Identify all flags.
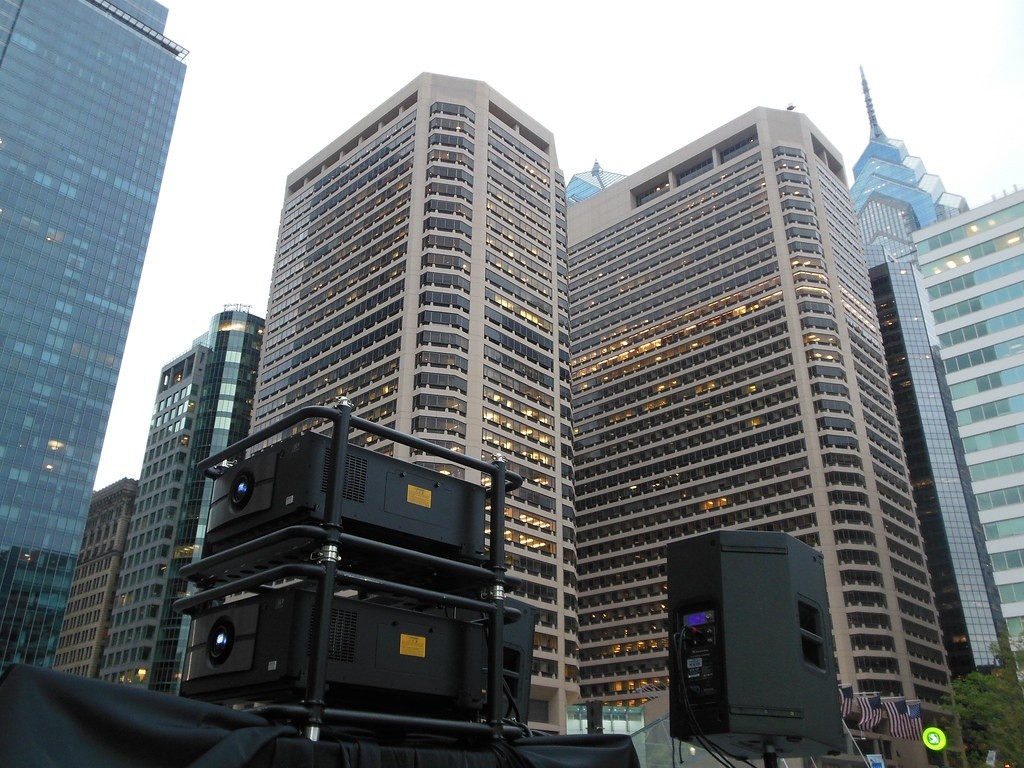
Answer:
[883,699,913,738]
[902,702,923,741]
[838,686,853,718]
[856,695,882,730]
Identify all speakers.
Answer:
[666,529,846,759]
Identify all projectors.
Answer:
[204,427,486,595]
[179,581,487,724]
